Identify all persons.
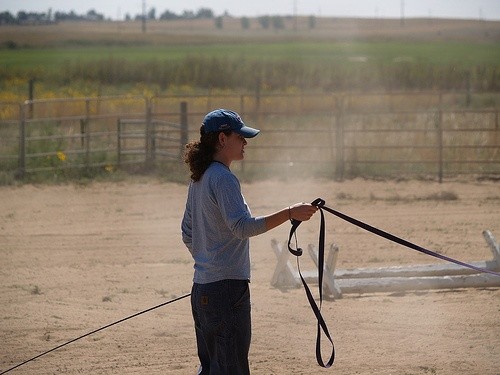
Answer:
[180,109,318,375]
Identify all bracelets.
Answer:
[287,206,293,219]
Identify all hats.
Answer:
[201,108,261,138]
